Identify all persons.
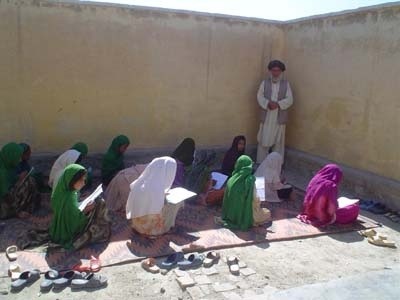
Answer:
[170,138,203,190]
[125,156,184,236]
[253,152,296,203]
[256,60,294,165]
[0,142,37,218]
[296,164,360,229]
[47,164,113,252]
[45,142,92,190]
[214,154,272,229]
[102,164,149,217]
[222,136,247,178]
[101,135,136,187]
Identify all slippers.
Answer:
[161,252,184,268]
[359,200,400,222]
[226,255,240,274]
[71,258,101,272]
[362,229,387,240]
[40,269,59,291]
[368,236,396,247]
[203,251,220,267]
[177,254,206,268]
[10,270,39,289]
[6,245,18,261]
[53,270,85,289]
[71,273,108,289]
[141,258,160,273]
[10,265,20,280]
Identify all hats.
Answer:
[268,60,285,72]
